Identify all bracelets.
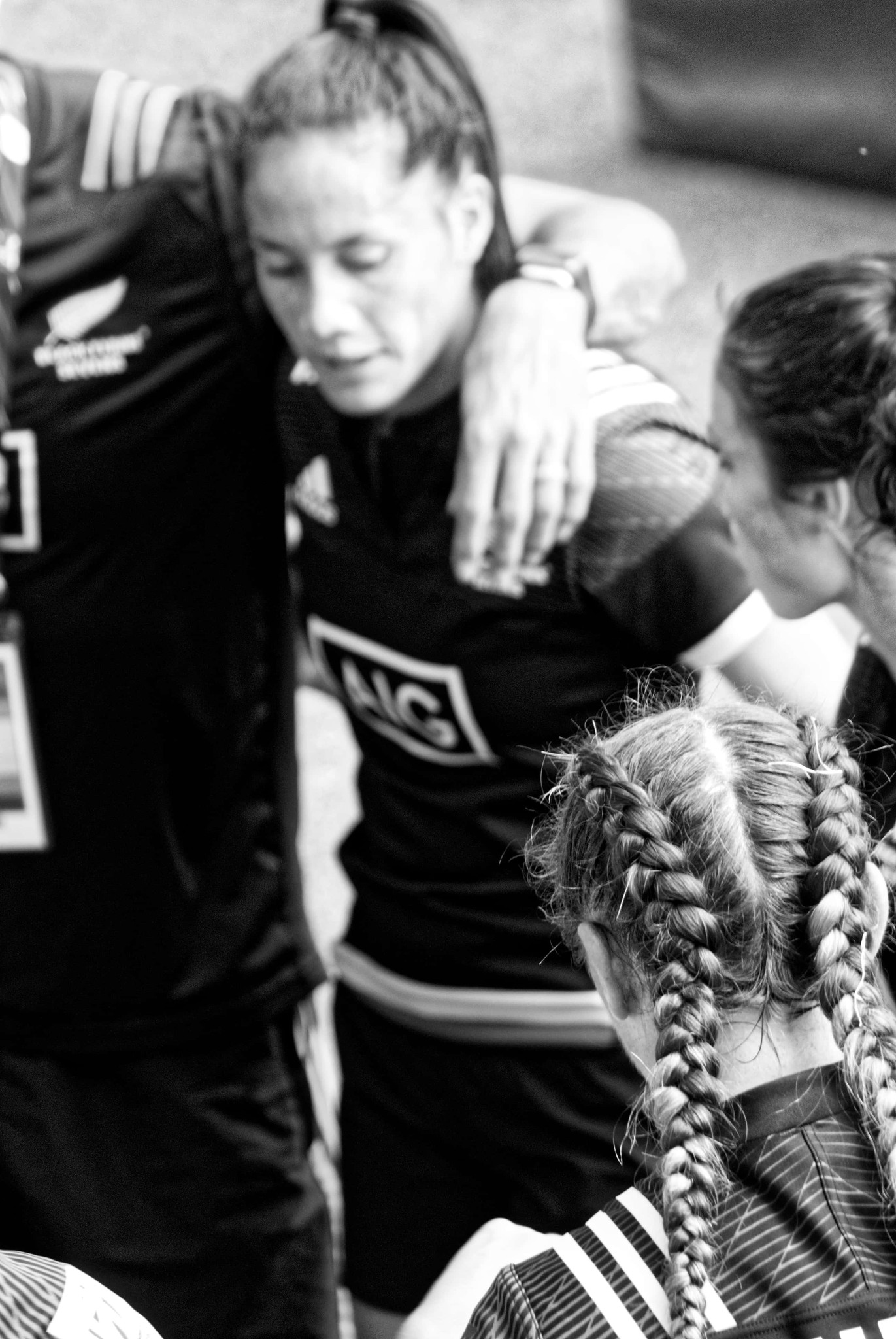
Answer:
[510,244,597,340]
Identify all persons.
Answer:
[232,0,848,1339]
[0,54,689,1339]
[708,255,896,829]
[451,696,896,1339]
